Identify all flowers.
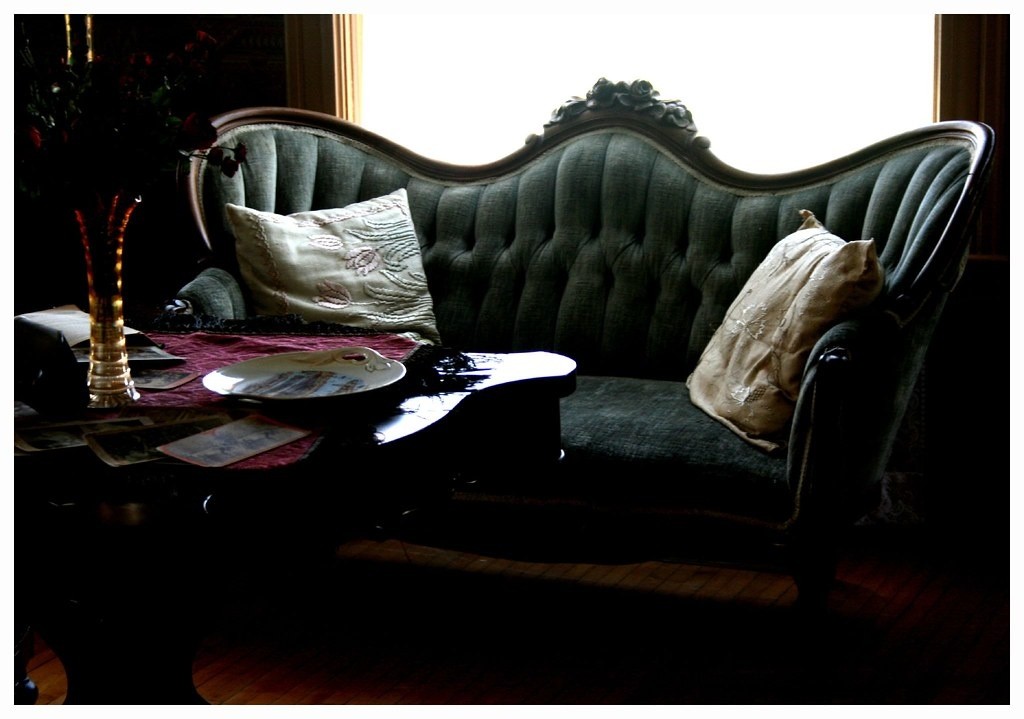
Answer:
[15,26,247,200]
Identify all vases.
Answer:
[69,193,147,407]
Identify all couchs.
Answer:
[147,79,996,705]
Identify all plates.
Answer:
[202,346,408,404]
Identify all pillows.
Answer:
[224,188,443,345]
[686,208,886,451]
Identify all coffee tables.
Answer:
[15,351,578,705]
